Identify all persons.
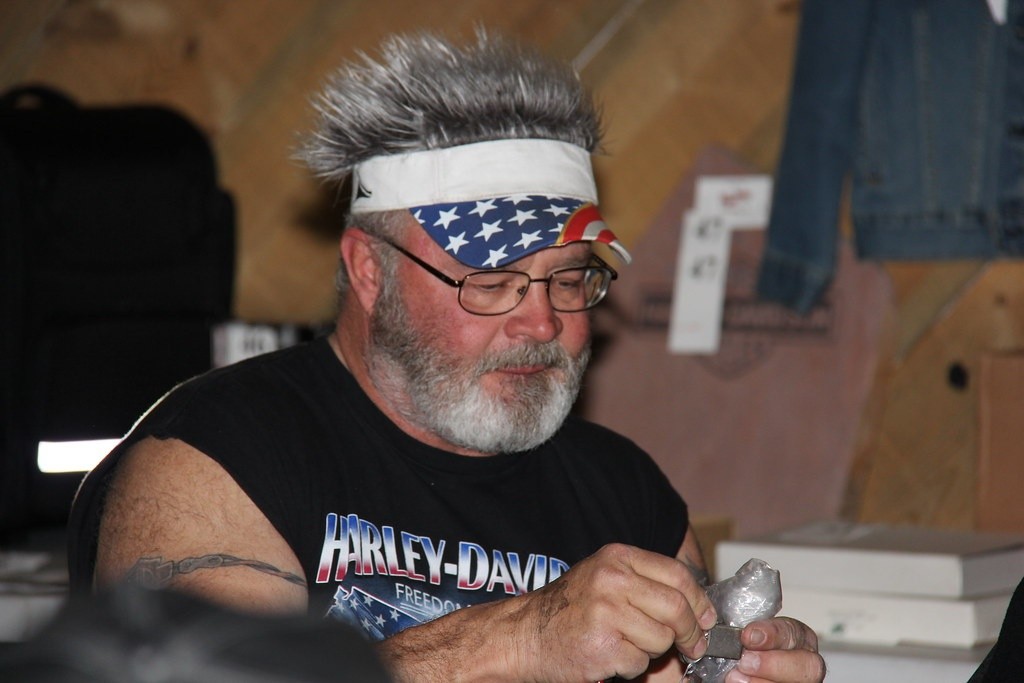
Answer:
[66,22,826,682]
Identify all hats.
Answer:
[349,136,633,271]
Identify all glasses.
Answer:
[356,225,619,317]
